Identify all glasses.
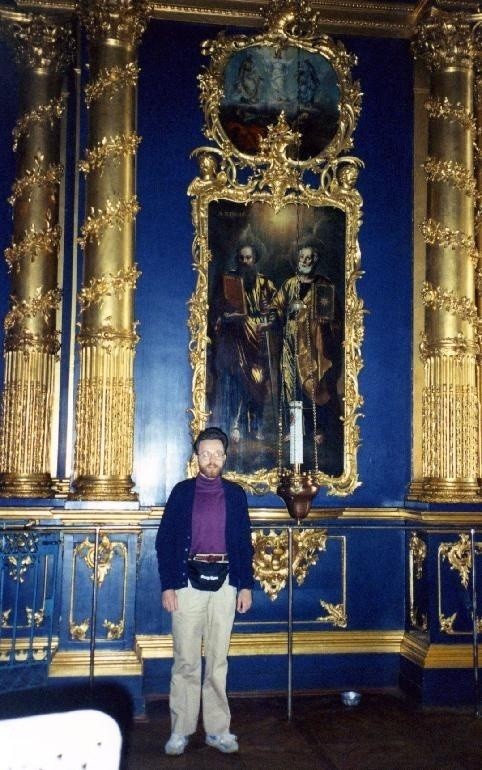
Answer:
[197,449,225,461]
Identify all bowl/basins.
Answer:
[341,690,362,706]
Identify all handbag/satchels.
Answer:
[185,559,229,591]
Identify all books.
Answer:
[215,270,247,313]
[298,281,336,320]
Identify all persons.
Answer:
[152,426,256,756]
[234,46,320,105]
[214,232,278,444]
[254,236,340,445]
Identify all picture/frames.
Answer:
[183,30,366,171]
[198,105,369,493]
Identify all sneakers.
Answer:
[204,732,241,755]
[165,733,190,756]
